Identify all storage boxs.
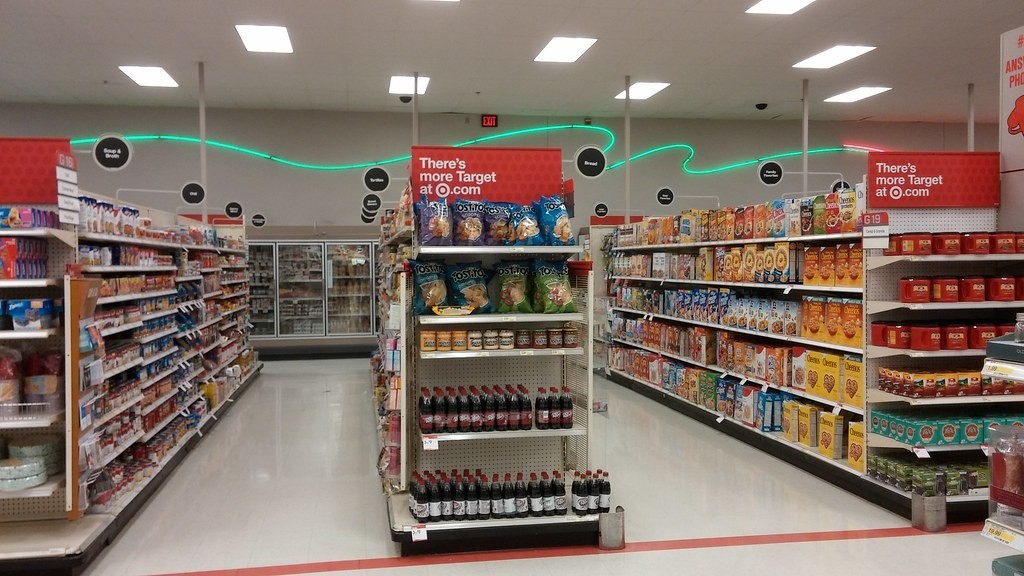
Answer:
[804,241,863,287]
[796,241,804,285]
[785,302,801,339]
[818,411,844,460]
[800,295,863,349]
[798,404,817,447]
[784,182,863,236]
[792,346,810,392]
[805,350,821,397]
[782,400,798,442]
[602,199,784,433]
[842,359,863,408]
[385,305,402,410]
[0,298,65,331]
[847,421,864,473]
[820,352,845,403]
[806,404,823,446]
[774,242,797,283]
[0,207,60,279]
[783,348,792,387]
[868,365,1024,497]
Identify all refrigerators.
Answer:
[253,237,379,356]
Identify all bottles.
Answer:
[571,469,612,516]
[491,472,567,519]
[408,467,489,525]
[419,384,532,434]
[536,386,572,429]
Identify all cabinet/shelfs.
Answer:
[328,256,370,317]
[0,204,117,576]
[598,232,867,496]
[280,258,322,322]
[386,245,602,558]
[979,358,1024,553]
[248,260,274,323]
[79,189,263,536]
[859,173,1024,526]
